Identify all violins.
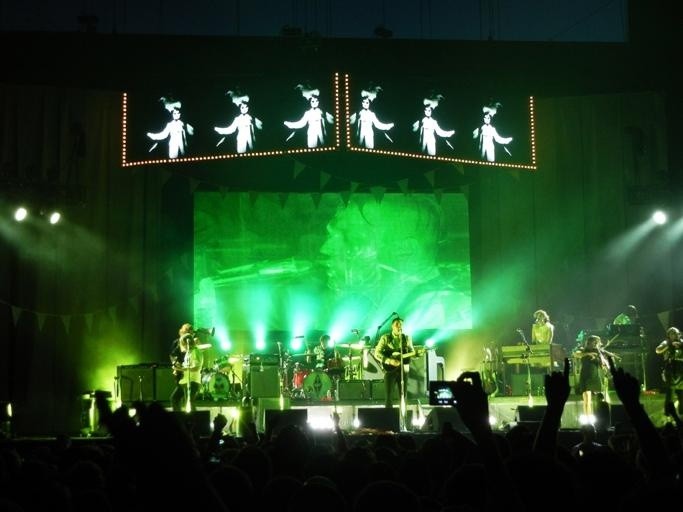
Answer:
[592,348,621,362]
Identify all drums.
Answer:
[326,359,344,373]
[294,367,311,390]
[212,357,232,375]
[200,368,216,383]
[201,372,230,401]
[302,372,335,401]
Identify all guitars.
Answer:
[381,345,438,372]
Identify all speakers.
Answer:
[371,380,399,402]
[425,408,470,433]
[339,380,371,401]
[358,409,399,432]
[611,404,644,423]
[170,411,210,436]
[607,348,647,391]
[117,363,177,406]
[251,353,280,402]
[266,409,307,436]
[516,406,547,421]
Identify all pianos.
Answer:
[501,344,561,374]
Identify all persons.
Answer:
[214,102,263,153]
[413,105,455,156]
[284,96,334,148]
[350,97,394,148]
[473,113,513,163]
[147,108,195,158]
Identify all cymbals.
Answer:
[341,343,362,348]
[342,356,361,360]
[294,353,318,356]
[195,344,211,349]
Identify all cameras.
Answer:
[429,381,471,406]
